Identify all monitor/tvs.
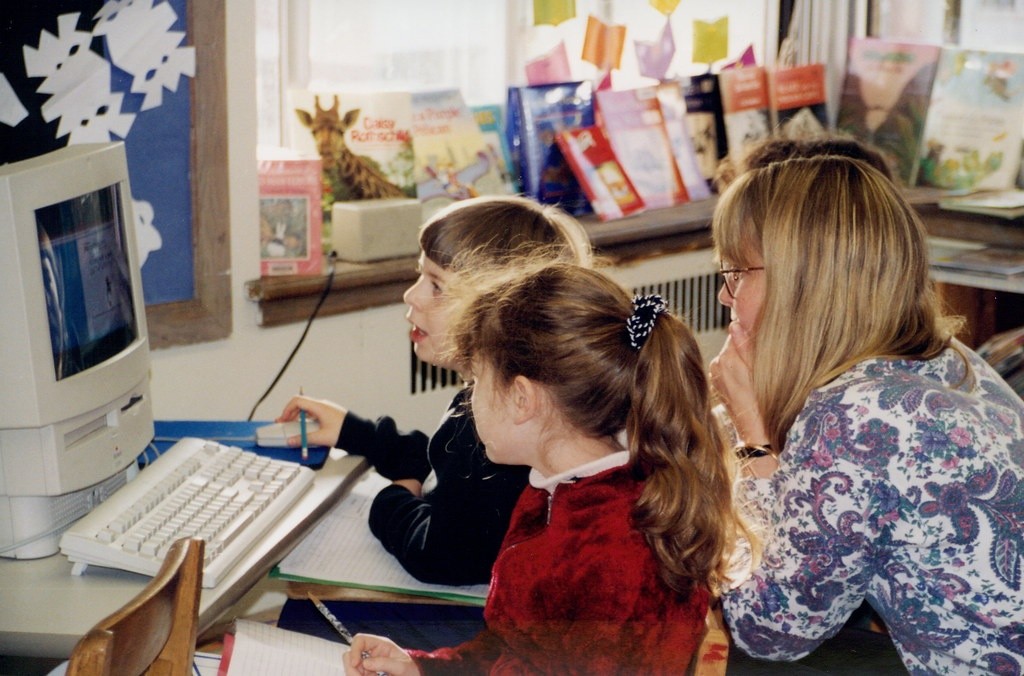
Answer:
[1,138,157,560]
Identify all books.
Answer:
[345,63,829,221]
[838,38,1024,279]
[271,473,492,606]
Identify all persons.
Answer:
[275,200,586,654]
[708,156,1024,675]
[745,140,891,180]
[343,264,758,676]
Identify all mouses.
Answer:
[255,419,322,449]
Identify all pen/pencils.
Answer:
[297,387,308,460]
[306,589,383,675]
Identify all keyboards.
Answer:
[59,435,316,592]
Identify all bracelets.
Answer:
[737,444,772,457]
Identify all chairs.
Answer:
[63,537,205,676]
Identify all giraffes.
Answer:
[292,95,410,202]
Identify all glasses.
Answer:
[720,259,764,299]
